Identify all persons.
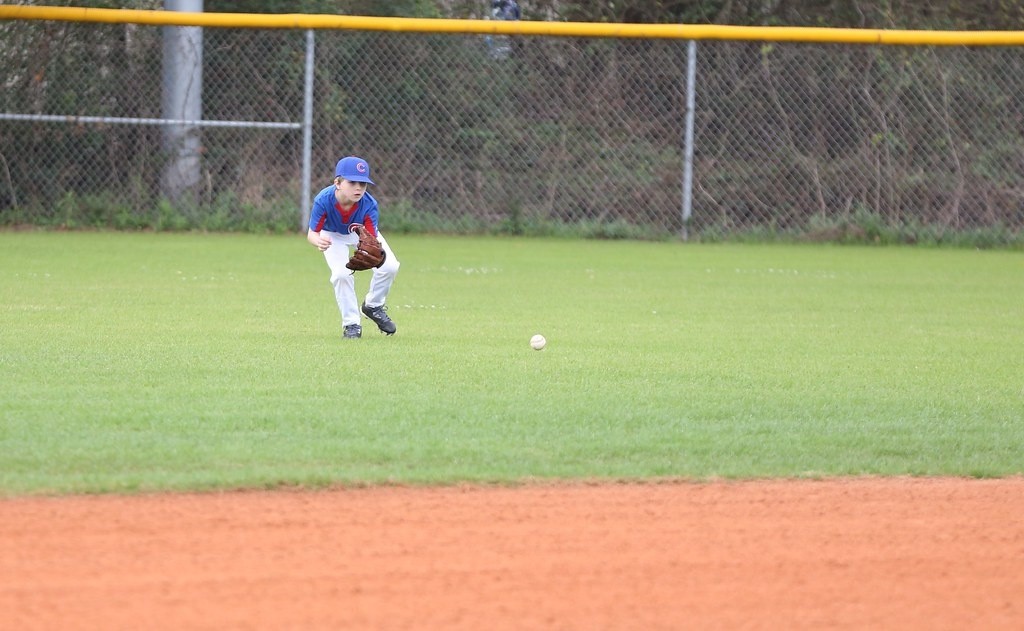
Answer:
[306,155,400,338]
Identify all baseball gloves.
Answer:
[344,225,387,272]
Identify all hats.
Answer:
[334,155,376,186]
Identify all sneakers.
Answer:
[343,324,362,339]
[360,300,396,336]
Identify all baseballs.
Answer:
[530,334,547,350]
